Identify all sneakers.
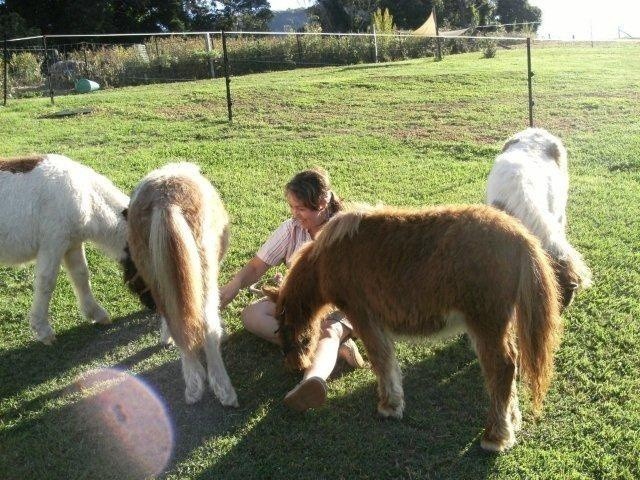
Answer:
[338,338,363,368]
[283,375,327,413]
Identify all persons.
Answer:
[219,170,364,413]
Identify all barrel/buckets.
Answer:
[75,79,101,93]
